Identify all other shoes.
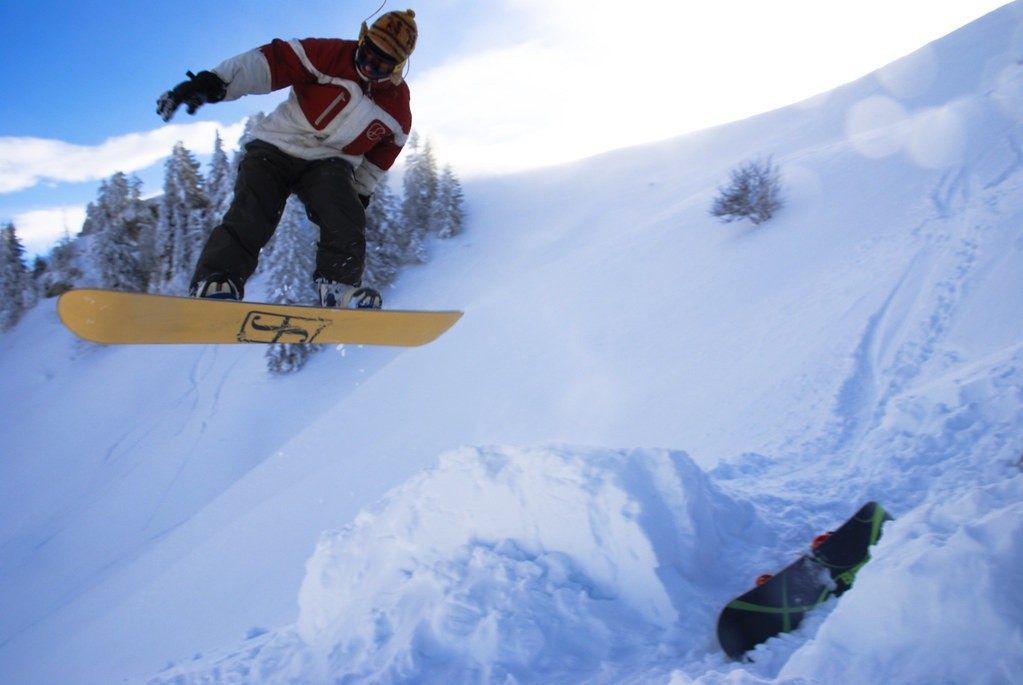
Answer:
[190,274,243,301]
[311,278,383,309]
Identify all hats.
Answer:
[359,9,418,73]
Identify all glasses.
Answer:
[355,47,393,79]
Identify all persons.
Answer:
[155,8,418,313]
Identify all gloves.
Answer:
[359,194,371,209]
[156,70,227,123]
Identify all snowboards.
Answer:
[57,287,462,347]
[718,499,896,665]
